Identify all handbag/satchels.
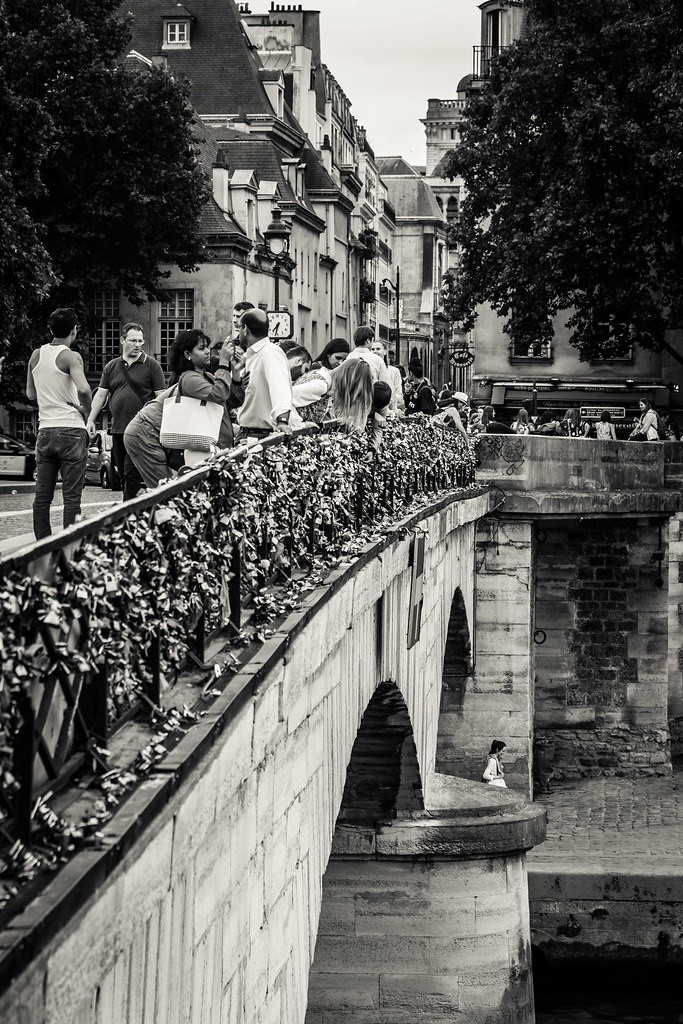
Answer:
[160,370,224,451]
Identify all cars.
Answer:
[84,429,114,490]
[0,432,62,483]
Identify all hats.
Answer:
[451,392,469,405]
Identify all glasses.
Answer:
[126,339,145,345]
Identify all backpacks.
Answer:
[650,412,668,441]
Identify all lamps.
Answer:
[667,382,679,393]
[625,379,635,387]
[550,377,561,385]
[479,379,492,388]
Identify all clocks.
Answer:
[265,310,294,340]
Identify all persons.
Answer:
[24,424,36,447]
[86,301,682,506]
[26,306,91,542]
[483,740,508,787]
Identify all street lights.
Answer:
[262,205,293,342]
[379,266,401,368]
